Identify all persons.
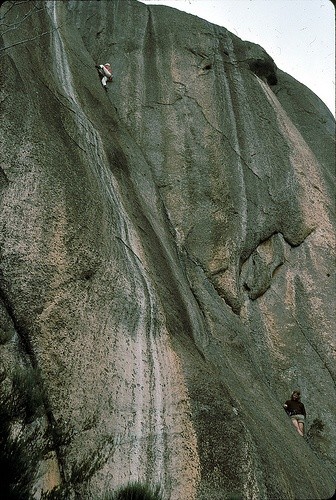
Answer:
[95,63,113,92]
[284,390,306,437]
